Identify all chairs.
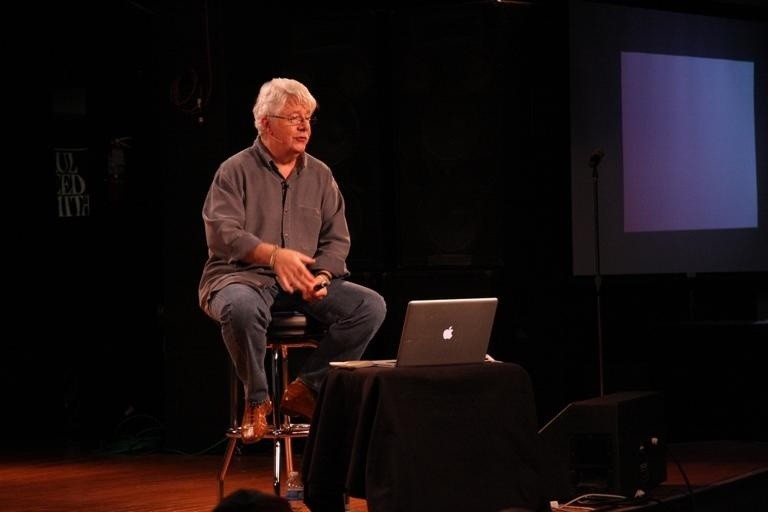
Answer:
[216,255,324,507]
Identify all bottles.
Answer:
[284,470,305,512]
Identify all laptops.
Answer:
[373,297,497,367]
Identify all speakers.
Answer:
[540,390,665,502]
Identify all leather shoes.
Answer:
[238,396,274,447]
[276,379,318,423]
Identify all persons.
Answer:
[196,74,388,445]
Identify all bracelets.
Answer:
[269,244,279,270]
[315,272,332,281]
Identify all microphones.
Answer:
[588,147,605,169]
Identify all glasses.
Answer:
[262,113,318,128]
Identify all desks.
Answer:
[317,358,537,510]
